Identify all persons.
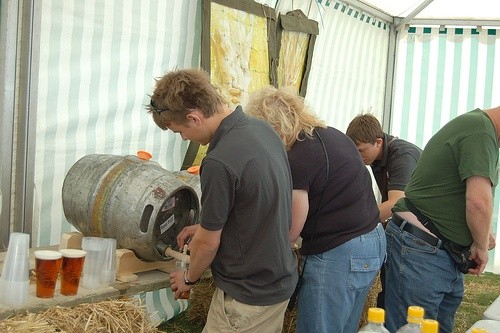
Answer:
[380,107,500,333]
[244,86,388,333]
[345,114,422,310]
[143,65,299,333]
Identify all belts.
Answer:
[392,213,445,250]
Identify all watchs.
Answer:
[183,269,200,286]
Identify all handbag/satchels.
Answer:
[444,242,477,274]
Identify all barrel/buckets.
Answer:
[61,152,202,262]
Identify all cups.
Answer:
[80,236,117,290]
[0,232,30,307]
[174,289,190,299]
[60,250,86,297]
[34,249,62,298]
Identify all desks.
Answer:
[0,246,213,322]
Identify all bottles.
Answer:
[395,305,424,333]
[421,319,439,333]
[357,307,390,333]
[470,327,489,333]
